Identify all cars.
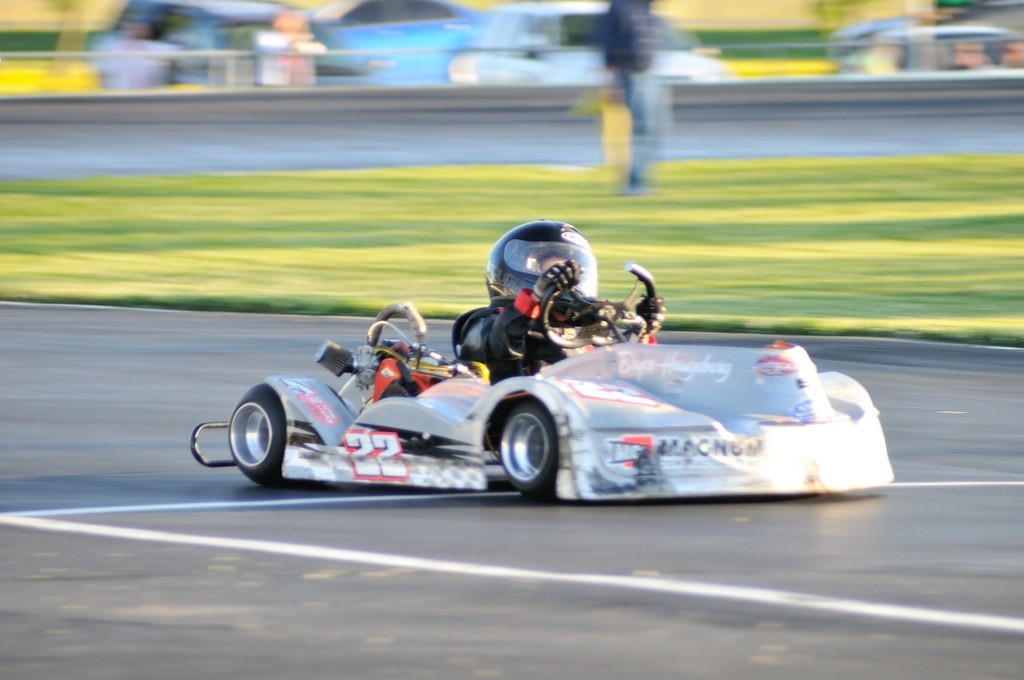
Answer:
[85,0,732,87]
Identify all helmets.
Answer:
[485,218,600,302]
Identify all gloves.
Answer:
[636,295,666,339]
[531,259,584,301]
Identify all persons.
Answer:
[254,11,326,86]
[605,0,671,195]
[452,220,666,386]
[89,9,182,90]
